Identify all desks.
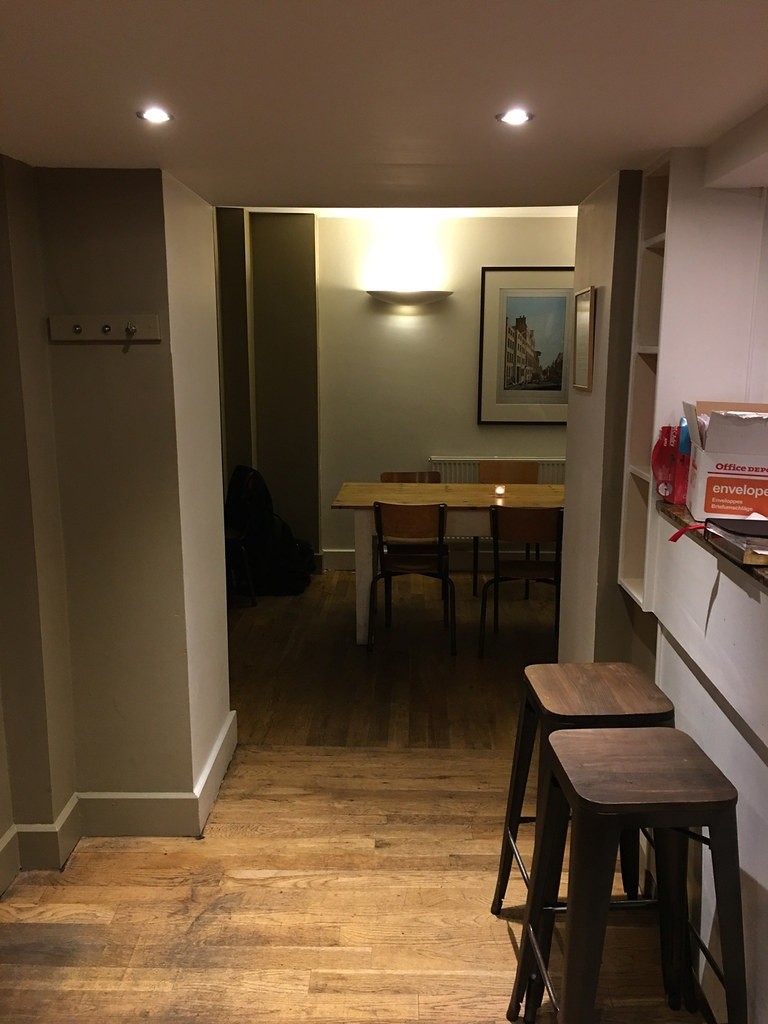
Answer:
[332,482,563,648]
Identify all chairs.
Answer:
[503,727,737,1024]
[480,503,567,645]
[466,459,544,600]
[365,501,450,650]
[491,659,678,937]
[369,470,446,623]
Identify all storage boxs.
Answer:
[687,402,768,521]
[652,426,687,504]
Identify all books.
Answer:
[704,517,768,565]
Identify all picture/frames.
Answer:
[571,285,595,392]
[477,267,573,425]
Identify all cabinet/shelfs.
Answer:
[618,195,666,612]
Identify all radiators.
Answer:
[428,455,566,542]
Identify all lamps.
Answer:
[356,285,460,307]
[134,106,174,128]
[492,101,530,132]
